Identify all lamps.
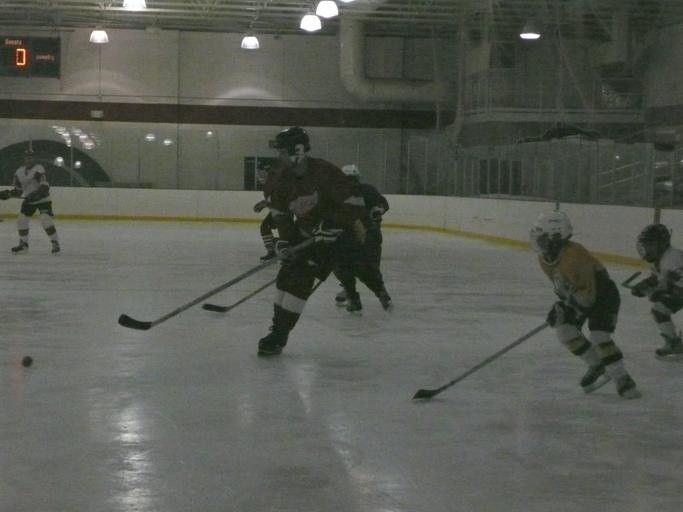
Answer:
[316,0,339,19]
[519,17,541,40]
[122,0,146,10]
[240,29,260,50]
[88,26,109,44]
[299,8,321,31]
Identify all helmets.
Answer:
[529,209,573,265]
[25,150,34,156]
[636,223,671,263]
[270,127,310,156]
[257,159,274,173]
[342,165,360,178]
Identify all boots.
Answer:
[257,302,300,354]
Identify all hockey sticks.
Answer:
[622,271,675,306]
[202,275,275,312]
[119,260,277,330]
[411,320,550,398]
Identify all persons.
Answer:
[253,159,294,260]
[11,148,61,253]
[257,127,369,354]
[334,164,391,311]
[529,209,637,401]
[631,224,683,360]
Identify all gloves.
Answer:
[0,190,12,199]
[27,191,39,202]
[254,200,267,212]
[547,300,578,327]
[632,280,651,298]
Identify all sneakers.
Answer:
[579,363,606,387]
[51,240,60,253]
[335,289,391,312]
[12,240,29,252]
[615,374,636,396]
[260,250,277,260]
[655,337,681,357]
